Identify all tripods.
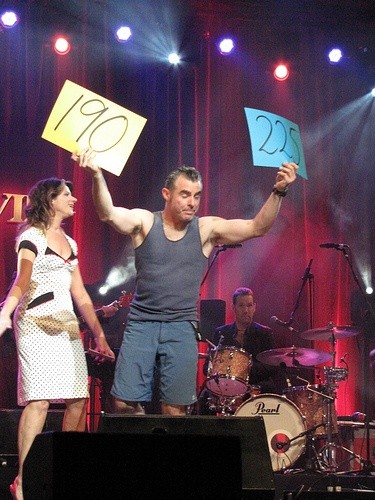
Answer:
[293,356,369,472]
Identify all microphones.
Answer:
[216,244,242,248]
[339,353,348,363]
[280,362,292,388]
[320,243,348,249]
[276,442,283,449]
[270,316,295,332]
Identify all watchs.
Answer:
[272,186,289,197]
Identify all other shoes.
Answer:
[10,476,19,500]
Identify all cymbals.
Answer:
[300,324,364,340]
[255,347,333,367]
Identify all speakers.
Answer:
[0,409,276,500]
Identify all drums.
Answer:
[283,382,339,438]
[216,384,258,416]
[204,344,251,399]
[324,368,346,381]
[233,392,306,473]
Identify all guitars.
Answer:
[76,289,134,326]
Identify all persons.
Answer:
[202,288,276,391]
[0,178,115,500]
[70,147,299,418]
